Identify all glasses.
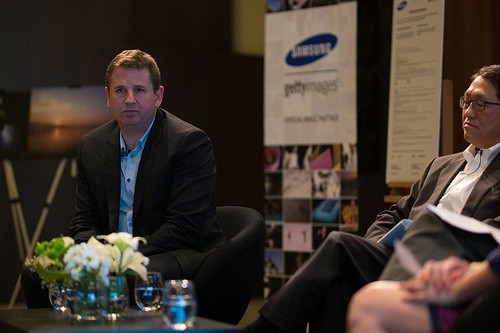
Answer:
[459,97,500,111]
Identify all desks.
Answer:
[0,308,244,333]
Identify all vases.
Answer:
[49,281,129,322]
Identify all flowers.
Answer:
[24,232,151,285]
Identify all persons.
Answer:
[347,246,500,333]
[68,50,226,309]
[223,65,500,332]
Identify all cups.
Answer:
[133,272,164,313]
[160,280,197,331]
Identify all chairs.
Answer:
[194,206,266,324]
